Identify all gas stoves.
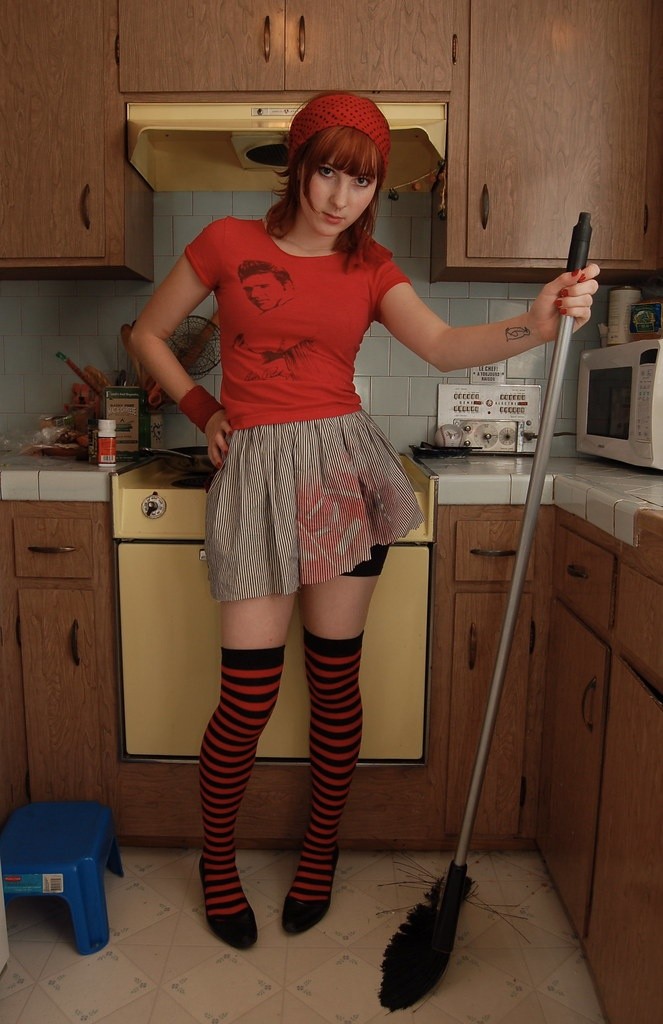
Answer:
[112,447,440,542]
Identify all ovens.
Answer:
[118,543,429,761]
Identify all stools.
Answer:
[2,800,126,956]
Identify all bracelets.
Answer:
[179,385,223,433]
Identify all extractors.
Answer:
[125,100,447,195]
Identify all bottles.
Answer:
[98,418,116,468]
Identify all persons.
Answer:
[130,90,599,950]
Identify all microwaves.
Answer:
[576,339,663,470]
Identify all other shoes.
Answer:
[195,855,257,951]
[282,847,339,933]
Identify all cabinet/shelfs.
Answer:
[430,0,663,288]
[1,499,662,1023]
[116,0,458,104]
[0,0,153,284]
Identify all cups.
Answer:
[149,413,165,448]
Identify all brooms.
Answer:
[377,206,594,1011]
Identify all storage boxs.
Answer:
[103,386,152,464]
